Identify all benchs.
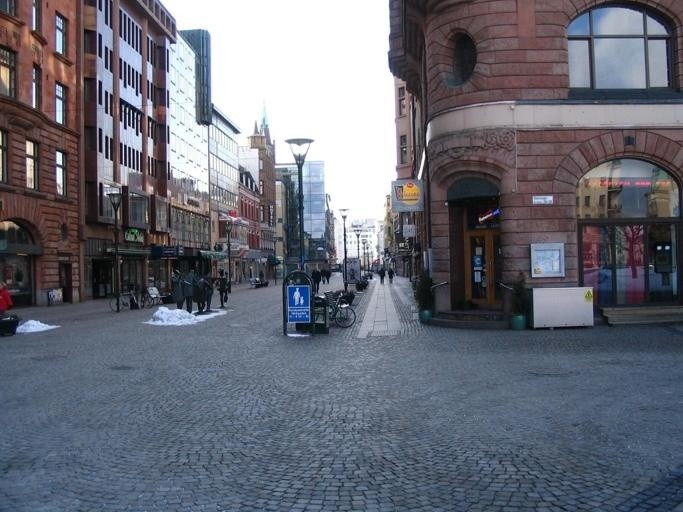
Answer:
[323,290,349,320]
[248,278,269,288]
[145,286,171,308]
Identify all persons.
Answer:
[172,267,229,313]
[259,270,265,287]
[378,268,395,285]
[310,265,331,294]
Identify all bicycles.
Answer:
[108,285,154,311]
[310,290,356,327]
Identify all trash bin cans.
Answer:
[94,282,106,297]
[309,295,329,334]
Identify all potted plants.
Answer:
[412,269,449,323]
[505,270,530,330]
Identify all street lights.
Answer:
[223,222,232,283]
[337,208,350,291]
[105,193,122,297]
[362,239,366,275]
[353,230,362,260]
[284,138,314,274]
[272,239,278,285]
[365,244,370,272]
